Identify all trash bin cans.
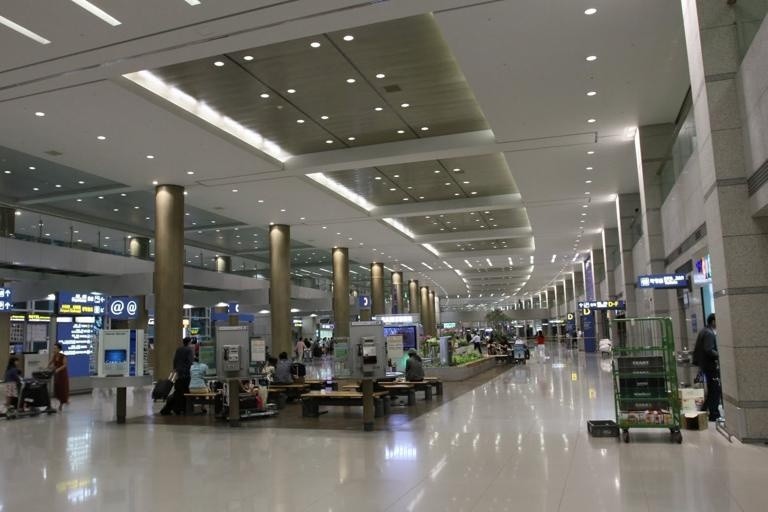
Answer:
[576,337,584,350]
[440,337,452,366]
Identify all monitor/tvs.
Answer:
[384,325,417,351]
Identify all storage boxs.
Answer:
[678,388,705,412]
[679,411,708,431]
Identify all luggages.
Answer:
[151,372,177,402]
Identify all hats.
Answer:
[408,348,417,353]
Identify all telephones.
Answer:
[223,345,240,362]
[356,337,376,356]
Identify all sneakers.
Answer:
[716,417,725,421]
[202,408,207,413]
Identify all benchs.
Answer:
[484,349,514,363]
[183,376,442,426]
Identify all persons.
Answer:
[566,327,581,349]
[44,342,69,413]
[533,331,546,365]
[466,330,524,355]
[404,348,424,382]
[293,337,332,362]
[5,357,21,386]
[692,312,724,422]
[173,336,294,417]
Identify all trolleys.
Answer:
[6,367,57,419]
[611,317,684,444]
